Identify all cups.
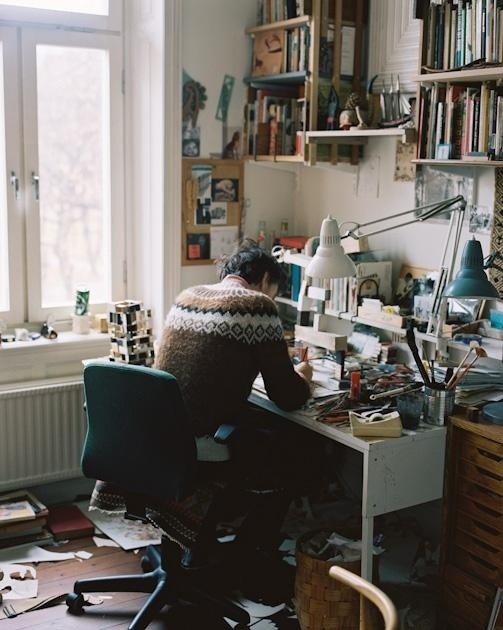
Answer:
[395,395,423,430]
[424,381,455,425]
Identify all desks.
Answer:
[249,371,447,629]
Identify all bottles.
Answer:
[74,286,89,315]
[255,218,288,249]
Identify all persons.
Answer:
[395,272,413,300]
[151,238,312,597]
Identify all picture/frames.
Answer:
[394,263,441,309]
[181,158,246,266]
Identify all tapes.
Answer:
[352,412,384,422]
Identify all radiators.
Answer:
[0,373,88,493]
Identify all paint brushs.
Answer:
[370,382,424,400]
[406,329,488,390]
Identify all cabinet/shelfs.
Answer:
[242,0,368,167]
[411,0,503,168]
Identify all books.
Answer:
[270,236,394,321]
[246,0,356,159]
[418,0,502,165]
[46,503,95,542]
[246,343,502,413]
[0,488,53,550]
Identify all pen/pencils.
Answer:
[302,347,308,361]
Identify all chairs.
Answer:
[66,364,286,630]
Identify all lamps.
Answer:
[442,235,503,301]
[305,196,467,338]
